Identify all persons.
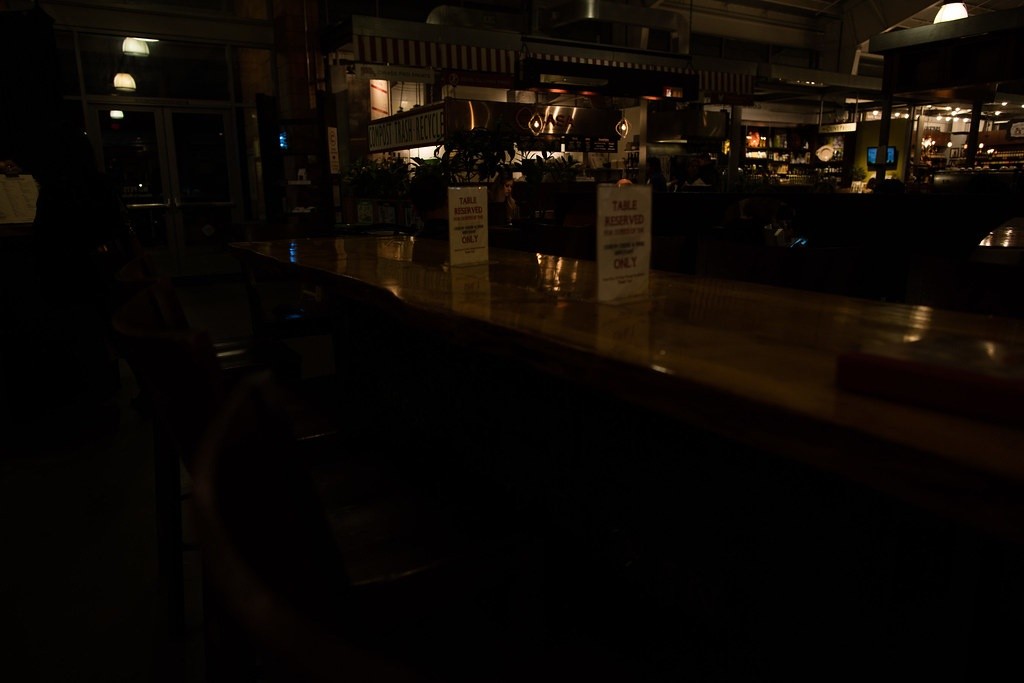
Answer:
[393,157,1024,308]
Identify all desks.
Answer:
[231,228,1023,481]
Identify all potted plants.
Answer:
[511,155,548,209]
[376,156,418,231]
[343,159,381,225]
[549,156,586,208]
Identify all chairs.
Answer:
[108,293,455,589]
